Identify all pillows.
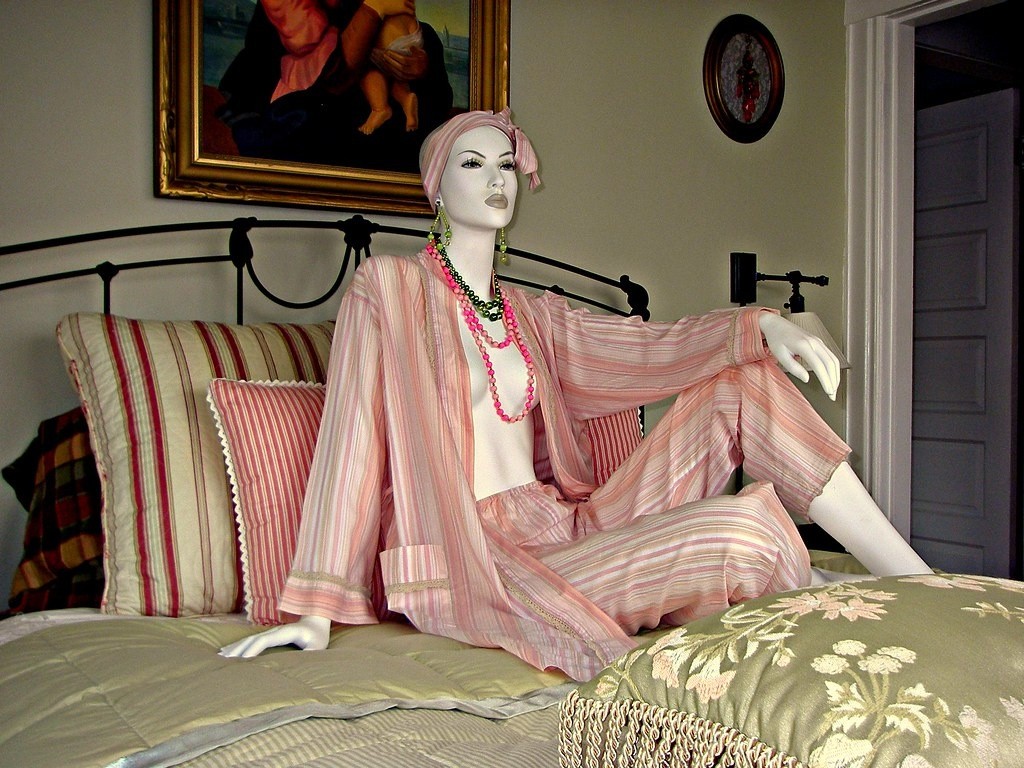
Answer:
[56,310,340,620]
[206,375,409,627]
[556,572,1024,768]
[581,404,645,491]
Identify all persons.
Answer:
[216,113,935,686]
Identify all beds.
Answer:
[0,215,848,768]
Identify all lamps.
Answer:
[728,252,854,375]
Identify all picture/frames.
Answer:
[702,13,785,144]
[151,0,512,220]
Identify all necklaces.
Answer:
[426,238,534,423]
